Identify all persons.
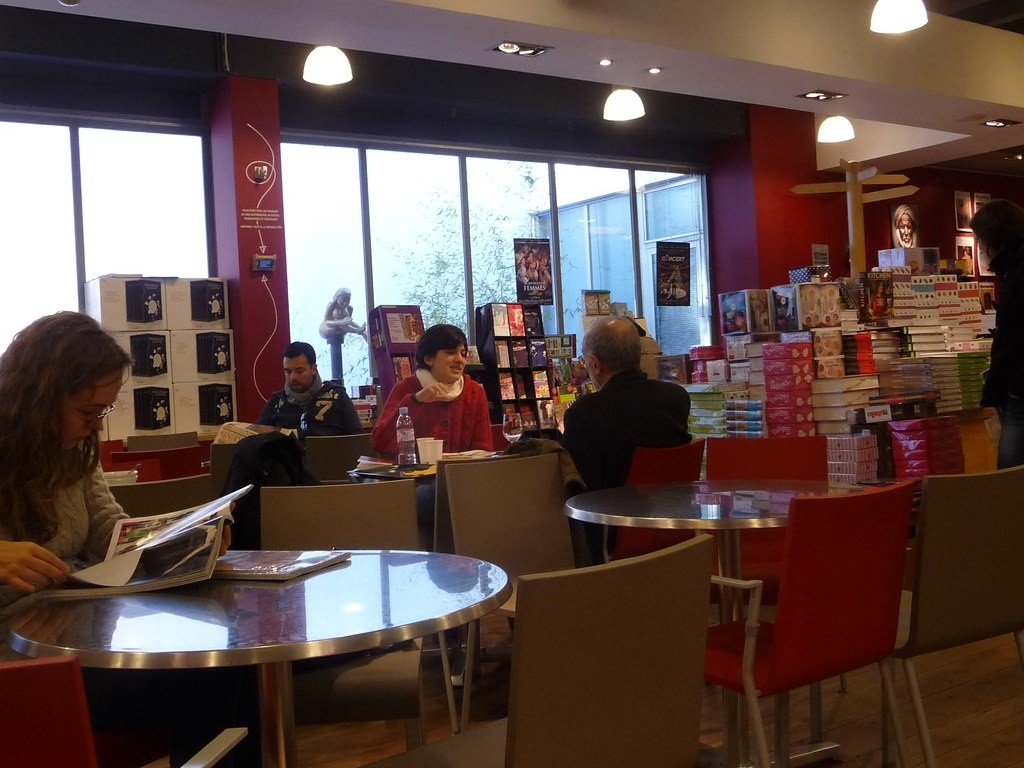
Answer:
[371,323,494,461]
[967,200,1024,470]
[0,311,262,768]
[562,316,693,566]
[868,279,892,316]
[962,246,973,275]
[256,341,364,446]
[318,287,367,341]
[515,245,551,284]
[893,205,919,249]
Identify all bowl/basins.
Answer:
[103,470,138,485]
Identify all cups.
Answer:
[502,413,523,444]
[416,437,434,464]
[425,440,443,464]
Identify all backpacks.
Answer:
[218,432,323,550]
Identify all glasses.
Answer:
[65,402,116,425]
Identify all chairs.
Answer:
[0,433,1024,768]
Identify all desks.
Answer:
[346,451,537,485]
[5,549,512,768]
[562,480,891,768]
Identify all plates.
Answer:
[442,449,497,457]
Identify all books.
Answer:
[211,550,351,581]
[42,484,254,597]
[835,271,894,324]
[743,324,992,434]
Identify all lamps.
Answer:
[870,0,929,34]
[603,84,646,122]
[817,113,855,143]
[302,44,353,85]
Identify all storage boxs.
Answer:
[654,354,708,383]
[765,373,815,392]
[877,463,965,481]
[718,282,843,334]
[107,384,175,442]
[788,265,832,284]
[173,381,238,433]
[84,277,167,331]
[169,330,235,382]
[877,426,959,441]
[879,435,962,453]
[763,341,813,360]
[688,345,722,361]
[105,331,172,385]
[850,414,959,435]
[878,448,964,463]
[768,421,817,437]
[763,358,815,376]
[164,277,229,331]
[766,406,814,424]
[764,389,811,406]
[877,455,965,469]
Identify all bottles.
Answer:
[396,407,416,465]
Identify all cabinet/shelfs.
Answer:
[473,301,560,435]
